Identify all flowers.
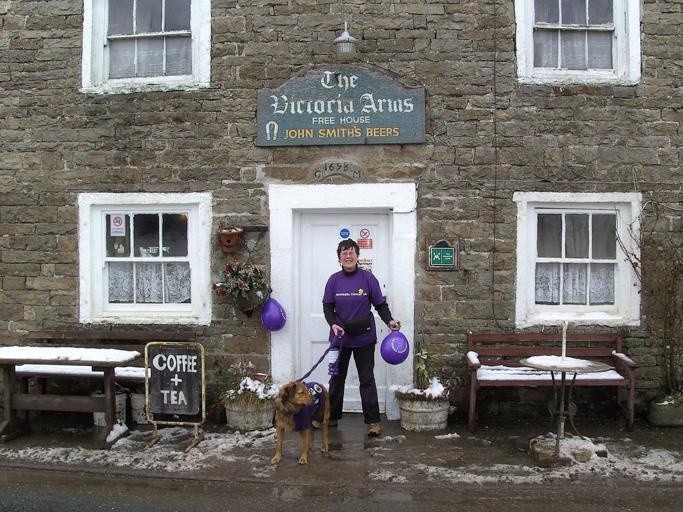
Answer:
[210,262,269,315]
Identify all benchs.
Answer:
[464,327,641,433]
[23,329,198,420]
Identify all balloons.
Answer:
[380,331,409,366]
[260,297,286,332]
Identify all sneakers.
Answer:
[327,419,339,426]
[366,424,383,436]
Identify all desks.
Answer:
[518,353,611,467]
[0,344,138,451]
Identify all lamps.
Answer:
[333,18,360,60]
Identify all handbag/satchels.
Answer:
[335,311,373,338]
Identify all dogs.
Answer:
[270,380,330,466]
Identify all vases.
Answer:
[216,223,242,253]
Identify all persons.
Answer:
[312,236,401,436]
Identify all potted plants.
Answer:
[396,336,461,432]
[213,357,274,430]
